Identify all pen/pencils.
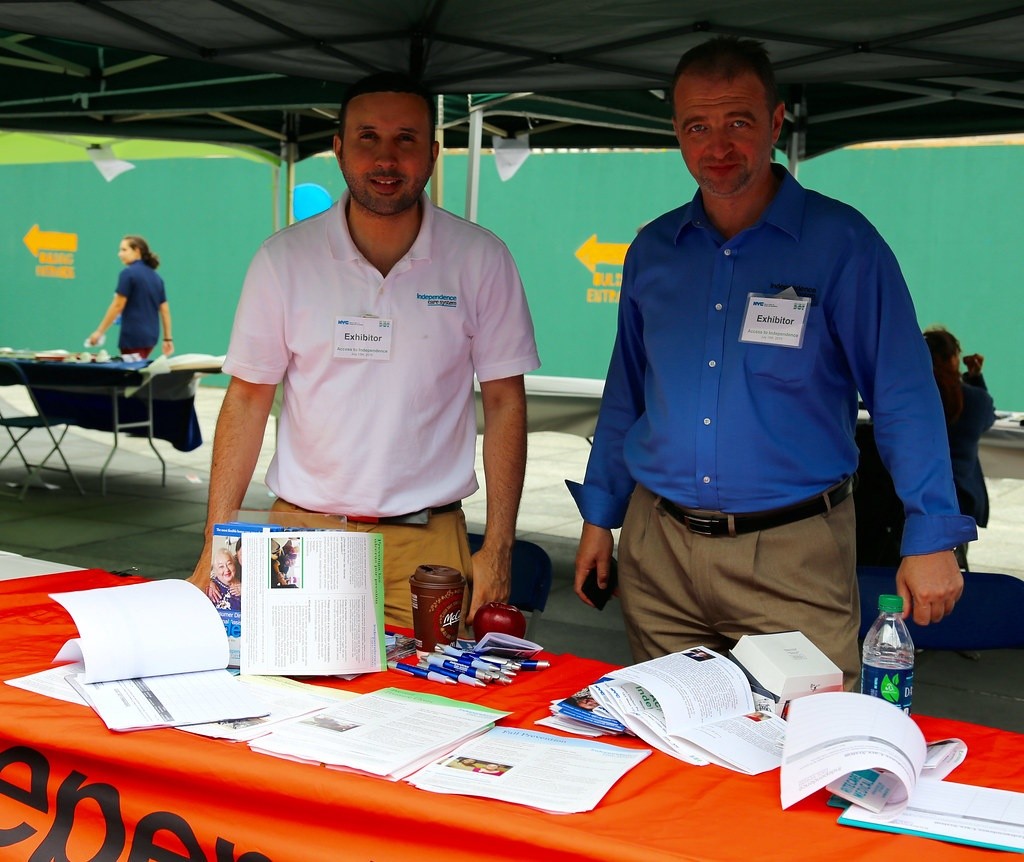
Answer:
[387,640,551,689]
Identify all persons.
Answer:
[185,72,541,636]
[576,39,977,693]
[89,235,174,360]
[922,330,995,529]
[210,538,242,609]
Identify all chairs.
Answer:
[856,565,1024,652]
[0,360,85,503]
[852,416,971,573]
[467,530,556,641]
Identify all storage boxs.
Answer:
[212,520,347,678]
[726,629,845,720]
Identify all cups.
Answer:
[410,563,467,659]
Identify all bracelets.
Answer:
[163,339,172,341]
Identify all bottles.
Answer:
[859,592,916,719]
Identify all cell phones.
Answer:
[582,556,618,611]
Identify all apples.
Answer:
[472,601,527,644]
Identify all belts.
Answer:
[651,479,854,537]
[378,498,463,526]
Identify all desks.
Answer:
[0,550,1024,862]
[0,356,223,497]
[471,374,1024,482]
